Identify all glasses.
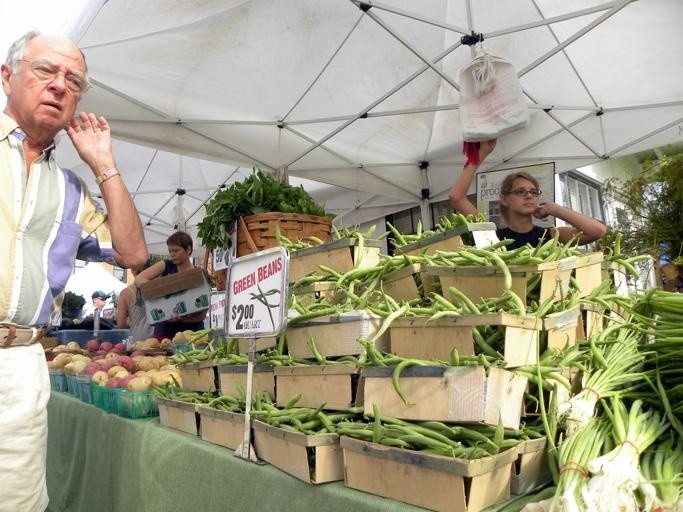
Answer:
[18,58,91,94]
[512,188,541,198]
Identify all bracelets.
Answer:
[95,168,120,184]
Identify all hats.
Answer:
[92,290,109,301]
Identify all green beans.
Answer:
[151,218,653,460]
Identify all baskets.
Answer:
[203,211,333,290]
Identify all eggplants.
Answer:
[47,315,116,335]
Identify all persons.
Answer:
[136,233,213,342]
[84,290,111,318]
[451,136,608,252]
[0,31,150,512]
[116,262,155,356]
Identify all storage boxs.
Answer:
[138,221,607,512]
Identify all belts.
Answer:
[0,322,49,351]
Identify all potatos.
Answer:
[45,331,210,416]
